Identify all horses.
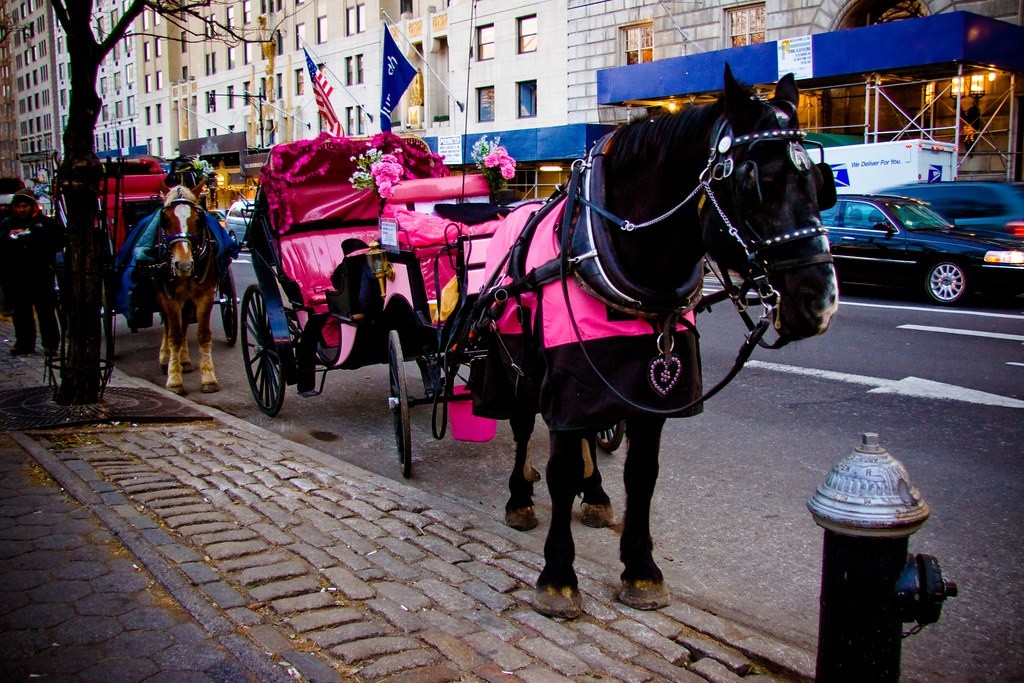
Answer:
[150,178,222,394]
[482,63,841,619]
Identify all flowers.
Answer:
[348,147,404,218]
[470,134,517,205]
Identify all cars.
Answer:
[818,194,1024,307]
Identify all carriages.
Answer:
[239,61,841,621]
[51,152,242,397]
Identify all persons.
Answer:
[1,188,67,357]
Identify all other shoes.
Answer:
[44,348,59,357]
[10,345,36,355]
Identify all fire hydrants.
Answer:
[805,432,960,683]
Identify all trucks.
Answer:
[804,139,957,197]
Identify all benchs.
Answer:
[276,173,491,315]
[95,172,168,257]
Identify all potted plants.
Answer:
[440,115,450,127]
[432,116,440,127]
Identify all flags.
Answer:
[380,20,419,135]
[302,47,345,138]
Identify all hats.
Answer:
[12,188,36,201]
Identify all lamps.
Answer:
[988,70,996,81]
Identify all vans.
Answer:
[225,200,257,250]
[873,180,1024,235]
